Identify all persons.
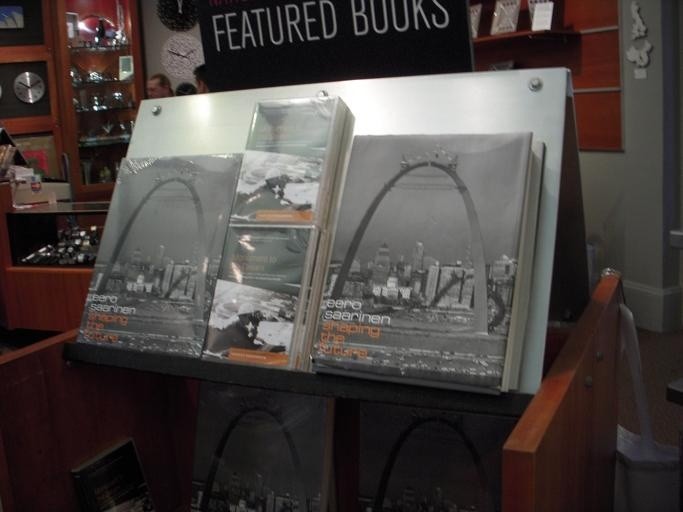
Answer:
[147,72,173,99]
[192,64,209,93]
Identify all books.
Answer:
[357,400,520,511]
[75,152,243,359]
[183,380,336,511]
[308,128,546,395]
[66,437,151,511]
[198,96,356,373]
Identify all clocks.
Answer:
[158,0,197,32]
[160,33,205,80]
[14,72,44,104]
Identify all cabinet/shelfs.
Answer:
[0,0,146,202]
[0,201,111,332]
